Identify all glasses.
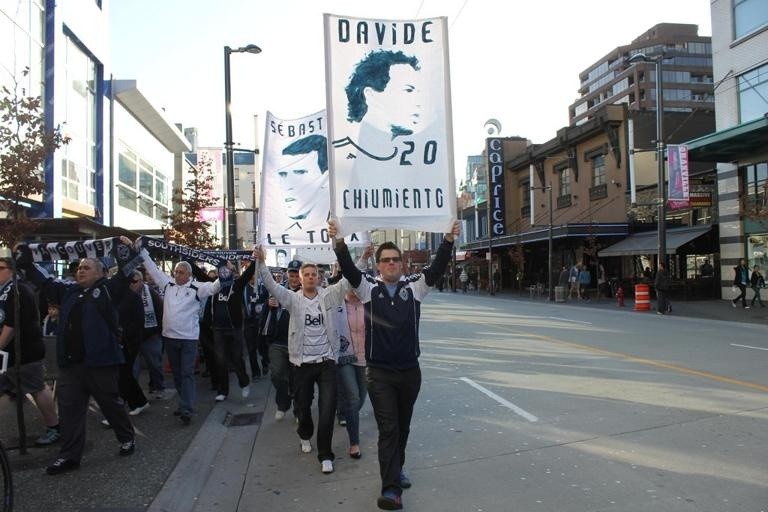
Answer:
[380,257,402,262]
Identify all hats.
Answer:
[287,260,301,271]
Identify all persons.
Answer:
[731,258,751,309]
[635,262,673,315]
[277,134,332,243]
[751,264,766,307]
[700,260,714,279]
[556,259,610,305]
[330,47,454,210]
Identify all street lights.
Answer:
[525,184,553,302]
[399,234,412,276]
[366,229,386,244]
[475,207,494,295]
[629,52,670,275]
[223,43,263,250]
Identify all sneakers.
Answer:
[275,410,284,420]
[300,420,361,472]
[153,365,269,422]
[378,465,411,510]
[34,402,149,474]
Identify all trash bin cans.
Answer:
[555,286,565,303]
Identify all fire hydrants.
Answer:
[614,288,624,307]
